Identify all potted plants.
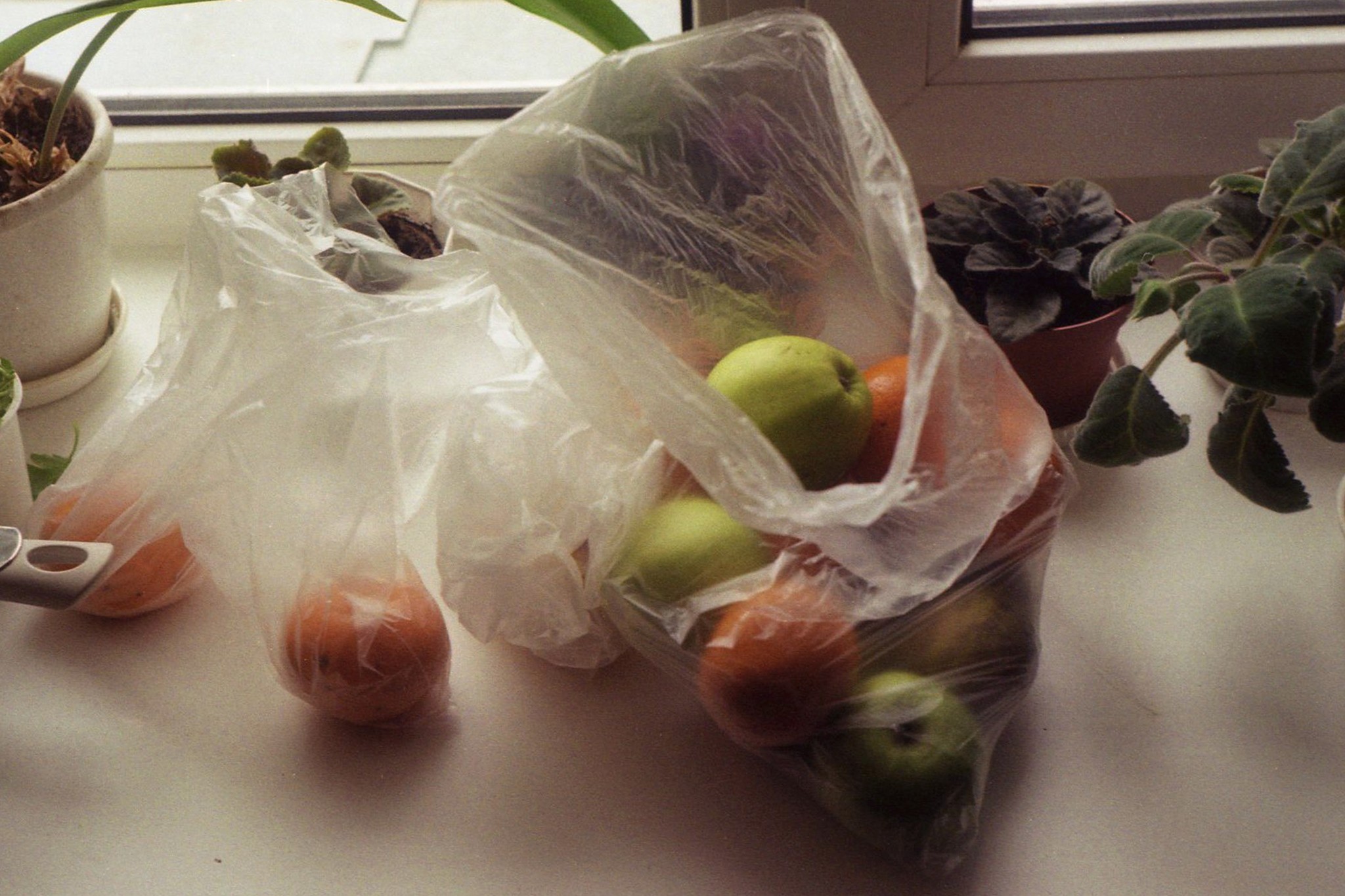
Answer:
[919,177,1155,429]
[0,355,36,542]
[1069,104,1345,515]
[0,0,652,411]
[211,125,454,259]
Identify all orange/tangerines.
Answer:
[888,572,1029,710]
[285,565,449,728]
[33,482,203,616]
[862,352,1023,499]
[697,577,856,753]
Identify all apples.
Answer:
[825,668,982,820]
[702,331,875,496]
[616,495,761,620]
